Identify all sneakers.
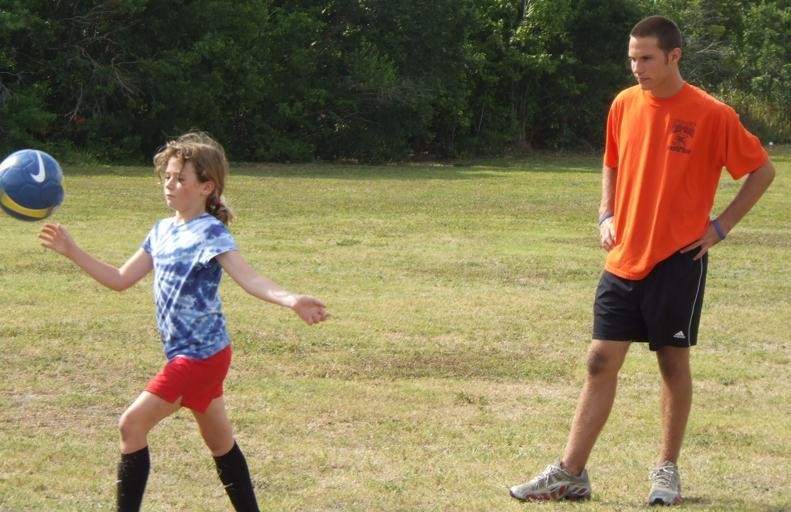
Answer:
[648,459,682,506]
[510,459,592,503]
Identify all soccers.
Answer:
[0,149,64,221]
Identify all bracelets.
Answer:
[712,219,725,240]
[598,211,614,225]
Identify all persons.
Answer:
[39,129,330,512]
[510,16,777,505]
